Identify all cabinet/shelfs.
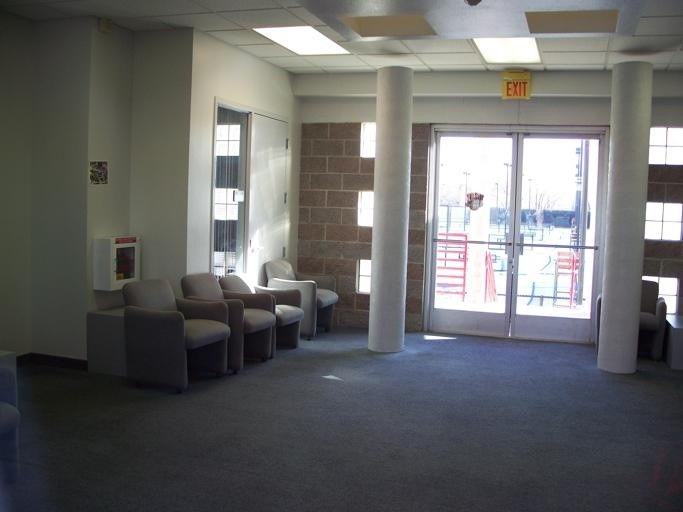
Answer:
[666,315,683,371]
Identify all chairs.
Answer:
[183,274,273,370]
[221,276,305,358]
[0,349,20,485]
[265,260,339,338]
[596,281,666,361]
[120,278,231,394]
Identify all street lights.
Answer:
[462,170,470,233]
[504,162,511,233]
